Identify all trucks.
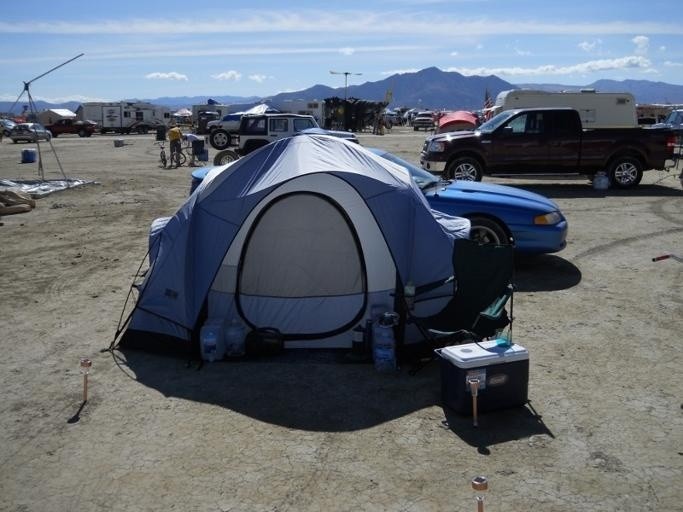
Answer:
[480,88,638,132]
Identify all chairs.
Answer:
[400,238,515,377]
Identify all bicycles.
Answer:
[171,144,203,165]
[152,140,167,168]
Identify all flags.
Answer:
[384,88,394,103]
[483,91,492,121]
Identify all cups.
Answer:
[495,327,511,347]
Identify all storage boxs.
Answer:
[432,339,530,418]
[21,146,36,164]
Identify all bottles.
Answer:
[351,324,365,355]
[223,317,246,358]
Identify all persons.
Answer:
[167,125,182,167]
[181,134,206,166]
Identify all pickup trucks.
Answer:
[420,107,675,190]
[134,118,170,135]
[42,118,99,137]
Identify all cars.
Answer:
[0,118,17,136]
[659,108,682,158]
[10,123,52,144]
[190,146,571,266]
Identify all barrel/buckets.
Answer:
[189,166,213,196]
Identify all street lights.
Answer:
[330,71,361,101]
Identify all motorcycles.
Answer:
[376,108,392,130]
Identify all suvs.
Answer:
[206,112,259,150]
[233,114,360,157]
[413,112,435,132]
[379,112,404,125]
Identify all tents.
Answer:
[100,134,470,373]
[431,110,481,136]
